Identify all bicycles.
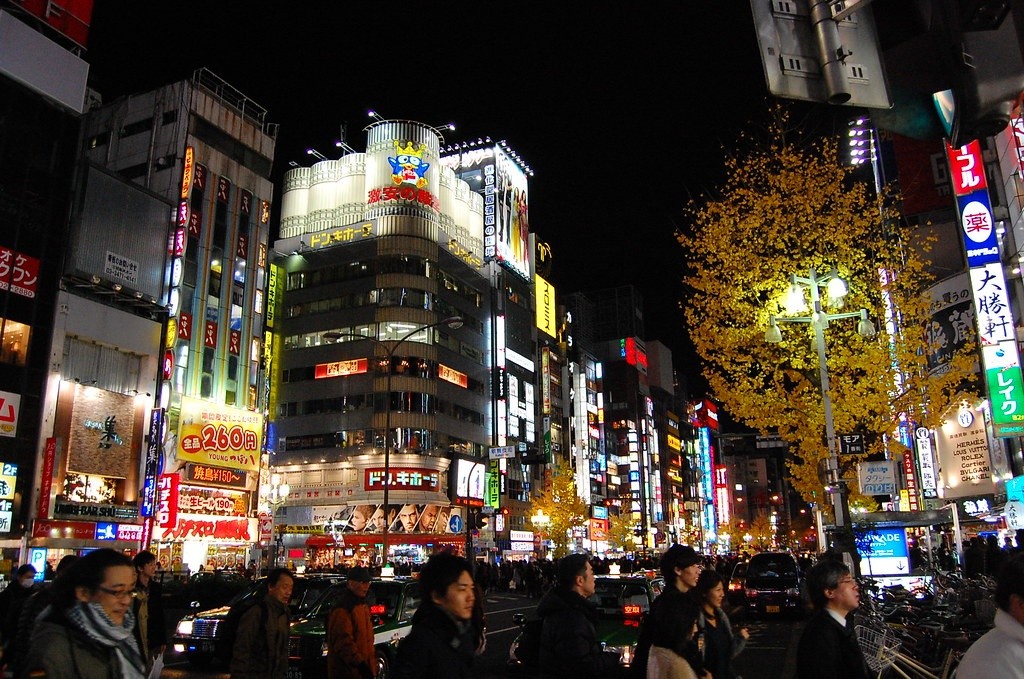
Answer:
[852,558,997,679]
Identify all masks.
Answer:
[22,579,35,588]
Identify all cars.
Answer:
[508,562,665,669]
[727,562,748,602]
[286,564,419,679]
[171,573,343,663]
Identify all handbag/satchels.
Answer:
[147,653,164,679]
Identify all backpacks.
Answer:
[215,593,290,660]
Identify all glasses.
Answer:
[360,581,372,585]
[840,579,858,586]
[99,586,138,600]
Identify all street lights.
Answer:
[767,268,876,527]
[323,316,465,569]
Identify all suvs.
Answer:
[745,550,805,618]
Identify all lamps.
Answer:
[489,272,502,278]
[150,303,173,312]
[458,251,474,257]
[129,296,158,306]
[335,140,356,153]
[73,275,100,287]
[477,261,492,267]
[367,110,384,120]
[364,227,377,236]
[95,284,122,293]
[306,148,327,161]
[117,292,143,301]
[330,235,340,242]
[436,122,534,176]
[300,240,305,252]
[436,237,456,247]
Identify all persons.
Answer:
[351,504,451,534]
[0,529,1024,679]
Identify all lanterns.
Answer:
[1004,498,1024,530]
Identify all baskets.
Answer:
[974,599,993,619]
[854,624,903,672]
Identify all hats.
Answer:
[663,544,702,572]
[348,566,374,583]
